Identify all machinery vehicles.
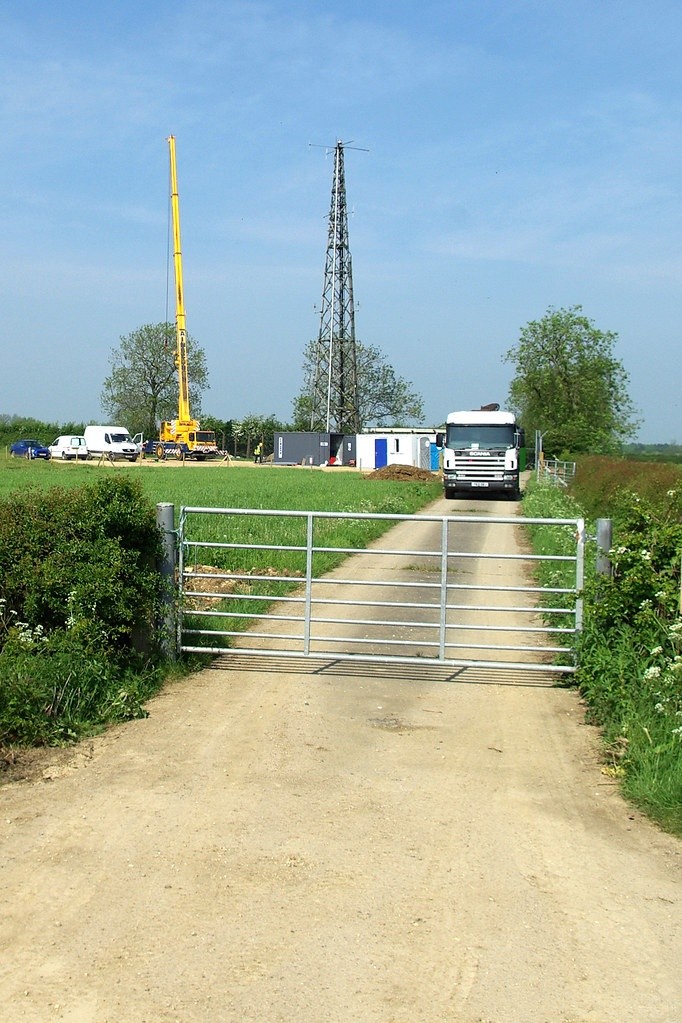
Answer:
[144,134,219,462]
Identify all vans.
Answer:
[47,435,88,461]
[83,426,143,462]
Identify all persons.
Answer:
[254,443,263,463]
[142,441,148,459]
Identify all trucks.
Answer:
[435,410,525,501]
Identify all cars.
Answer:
[9,439,51,460]
